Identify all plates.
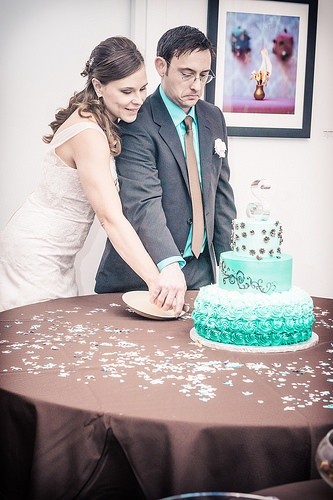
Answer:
[122,290,189,319]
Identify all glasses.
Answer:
[166,59,216,85]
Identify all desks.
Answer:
[0,289,333,500]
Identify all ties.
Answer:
[183,115,205,259]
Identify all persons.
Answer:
[94,25,237,318]
[0,36,181,314]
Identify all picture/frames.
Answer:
[204,0,318,138]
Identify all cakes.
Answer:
[191,217,315,346]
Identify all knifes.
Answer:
[184,304,195,314]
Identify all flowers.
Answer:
[214,138,227,158]
